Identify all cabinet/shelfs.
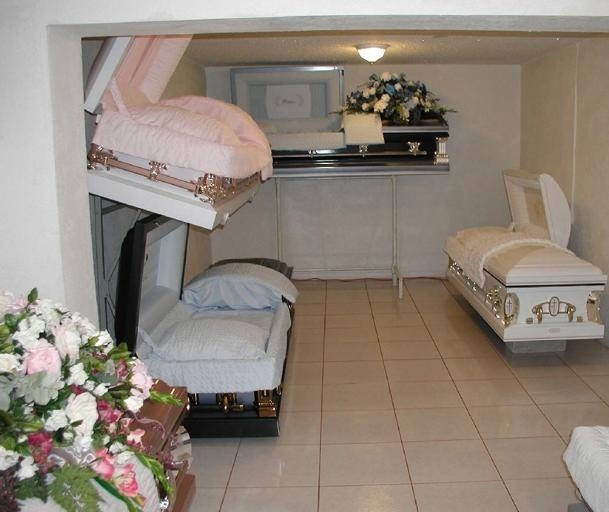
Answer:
[128,376,198,511]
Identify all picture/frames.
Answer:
[233,69,342,135]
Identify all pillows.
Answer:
[181,261,301,312]
[158,94,270,150]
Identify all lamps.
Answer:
[352,43,392,65]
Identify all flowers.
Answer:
[0,283,186,511]
[328,71,460,126]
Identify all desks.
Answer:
[269,163,451,299]
